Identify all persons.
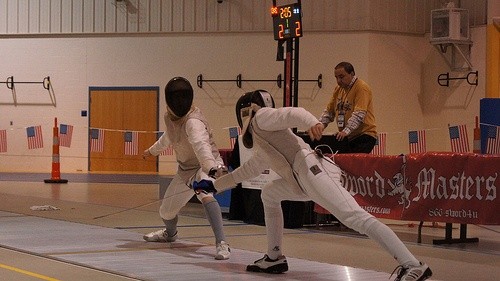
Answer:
[318,62,379,153]
[143,76,232,260]
[193,90,433,281]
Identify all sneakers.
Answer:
[389,263,433,281]
[143,230,178,242]
[246,254,289,273]
[214,240,231,260]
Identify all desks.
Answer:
[314,152,500,246]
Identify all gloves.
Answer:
[207,167,228,180]
[196,179,218,194]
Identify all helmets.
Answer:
[165,77,193,118]
[236,89,275,150]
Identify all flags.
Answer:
[124,130,139,156]
[408,130,426,153]
[58,124,73,148]
[0,130,7,153]
[229,126,243,150]
[26,125,44,150]
[156,131,174,156]
[449,125,470,153]
[486,124,500,155]
[371,132,386,156]
[91,128,105,153]
